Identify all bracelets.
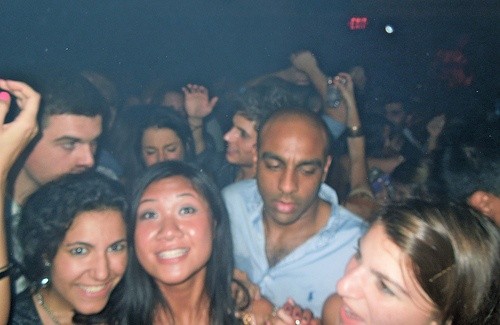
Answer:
[190,124,202,130]
[0,262,14,280]
[344,127,363,137]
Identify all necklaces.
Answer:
[37,293,64,325]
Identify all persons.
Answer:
[0,49,500,325]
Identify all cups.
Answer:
[325,75,347,108]
